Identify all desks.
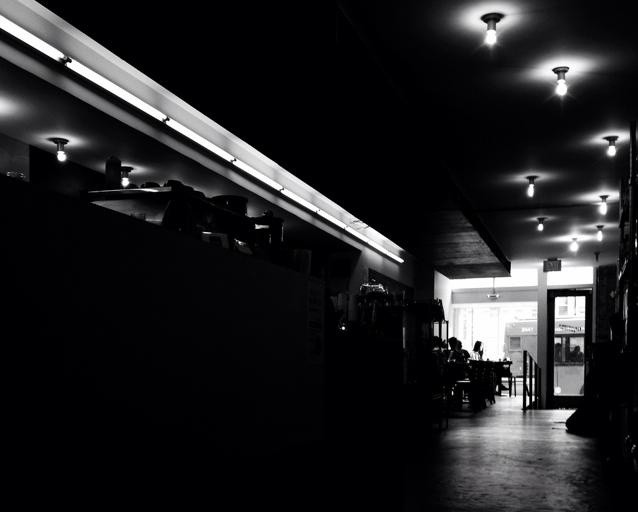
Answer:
[492,361,513,397]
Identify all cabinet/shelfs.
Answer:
[407,298,450,430]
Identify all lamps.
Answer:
[480,11,619,252]
[120,170,131,187]
[57,142,68,162]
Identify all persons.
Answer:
[443,336,583,399]
